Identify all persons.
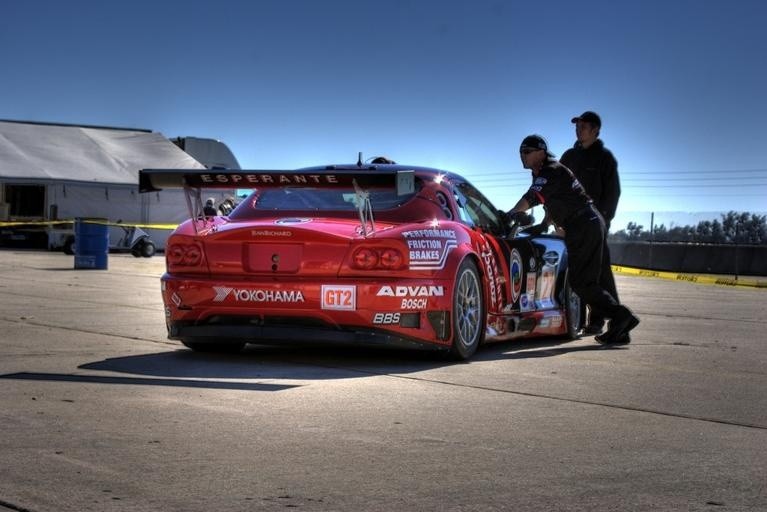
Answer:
[198,195,243,217]
[497,134,638,346]
[559,111,619,334]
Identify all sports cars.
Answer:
[133,150,599,361]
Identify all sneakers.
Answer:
[583,324,603,336]
[595,311,642,347]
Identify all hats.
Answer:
[570,110,602,127]
[520,134,556,158]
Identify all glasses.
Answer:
[519,149,541,155]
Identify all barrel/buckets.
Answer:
[73,216,110,270]
